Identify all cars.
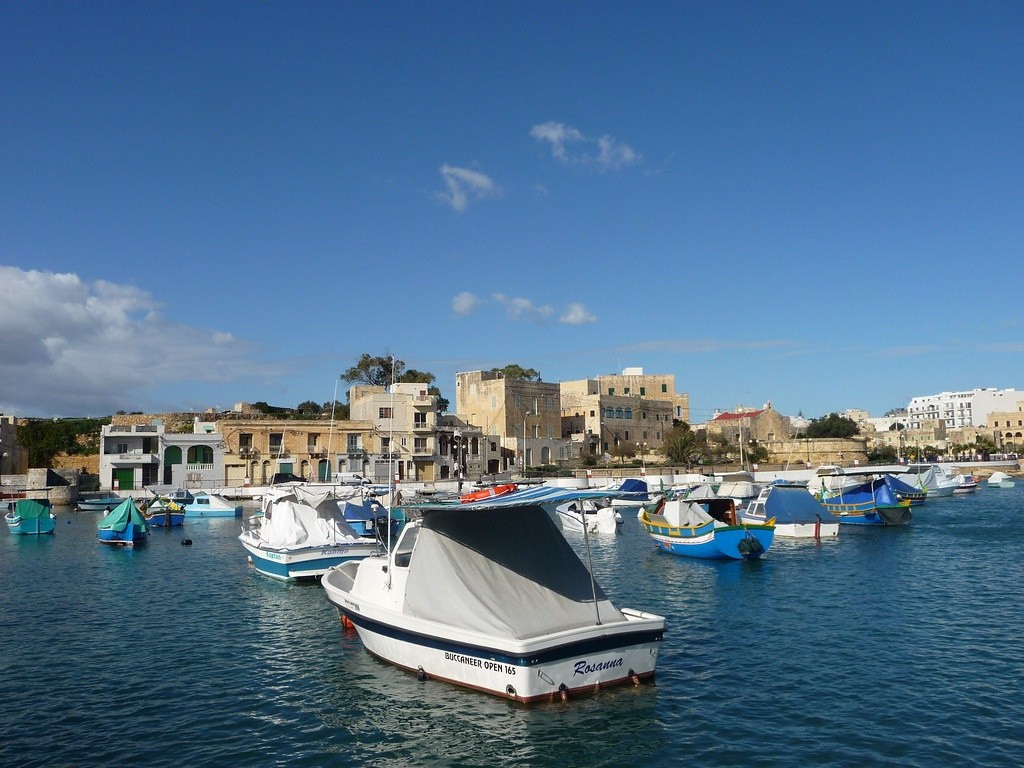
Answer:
[336,473,370,485]
[269,473,309,486]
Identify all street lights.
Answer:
[636,442,649,467]
[523,412,530,478]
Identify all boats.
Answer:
[951,474,977,494]
[458,483,516,503]
[806,462,927,526]
[186,494,243,517]
[76,498,136,511]
[158,488,195,504]
[97,496,150,546]
[898,465,960,497]
[5,482,57,535]
[555,499,624,535]
[593,469,699,506]
[637,492,777,560]
[322,353,668,704]
[739,474,840,537]
[138,495,186,527]
[234,422,400,582]
[987,472,1015,488]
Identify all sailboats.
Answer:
[716,420,763,498]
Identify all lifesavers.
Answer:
[461,483,516,502]
